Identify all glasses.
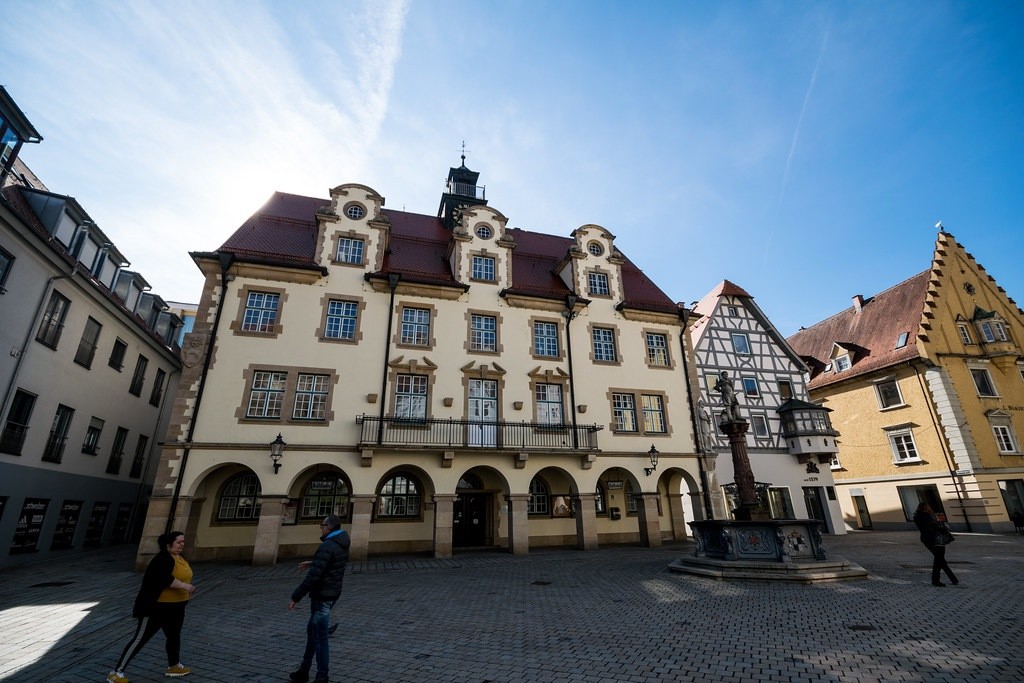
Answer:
[320,522,328,529]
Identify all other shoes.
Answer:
[953,580,959,585]
[936,582,946,587]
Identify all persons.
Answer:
[714,370,746,421]
[912,501,961,587]
[1012,510,1023,533]
[106,530,196,682]
[286,513,351,682]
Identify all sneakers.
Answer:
[312,669,329,683]
[289,669,309,683]
[107,671,128,683]
[165,661,191,677]
